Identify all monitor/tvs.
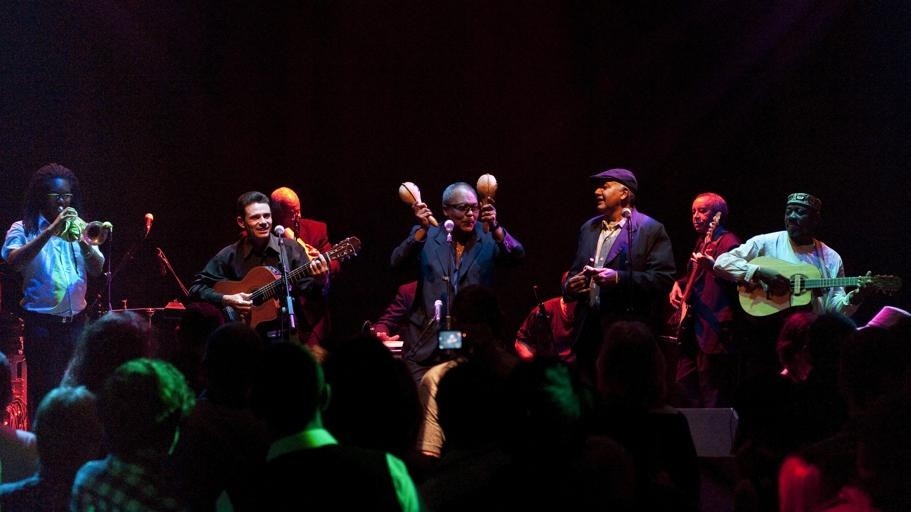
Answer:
[438,329,467,356]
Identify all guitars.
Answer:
[211,237,362,330]
[669,211,723,338]
[738,255,903,319]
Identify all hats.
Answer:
[856,304,911,332]
[589,168,638,196]
[786,192,821,212]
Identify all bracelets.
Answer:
[490,219,502,233]
[613,269,623,286]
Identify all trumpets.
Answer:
[58,207,109,245]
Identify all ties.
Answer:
[455,240,464,271]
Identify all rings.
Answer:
[698,260,703,266]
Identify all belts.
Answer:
[22,308,85,325]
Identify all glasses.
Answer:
[46,191,73,200]
[445,205,480,211]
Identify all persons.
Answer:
[1,163,103,425]
[732,307,911,512]
[72,298,265,512]
[509,267,583,368]
[669,192,742,407]
[1,354,40,483]
[435,284,587,512]
[215,342,425,512]
[581,320,701,510]
[559,166,679,377]
[368,275,473,464]
[187,192,328,334]
[710,190,883,349]
[270,187,341,347]
[0,385,102,512]
[387,180,526,332]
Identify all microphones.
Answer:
[144,212,155,237]
[433,299,443,324]
[274,223,288,260]
[443,219,455,243]
